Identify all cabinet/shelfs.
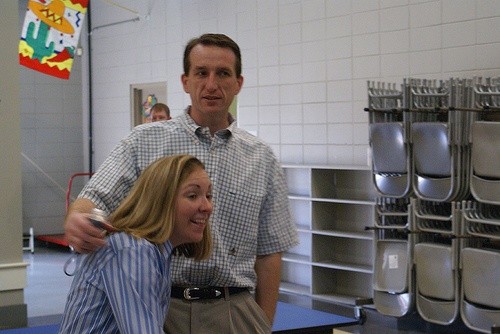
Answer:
[281,163,376,313]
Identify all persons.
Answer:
[65,34,298,334]
[59,155,217,334]
[149,103,172,122]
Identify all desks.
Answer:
[0,300,359,334]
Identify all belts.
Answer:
[170,286,249,301]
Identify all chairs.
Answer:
[365,73,500,334]
